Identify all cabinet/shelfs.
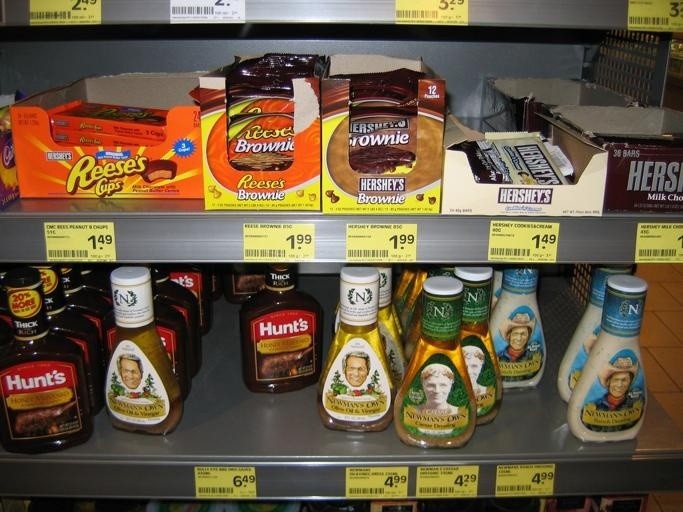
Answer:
[0,1,683,501]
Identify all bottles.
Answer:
[567,274,648,442]
[103,266,183,436]
[395,268,456,364]
[62,263,114,339]
[239,262,324,395]
[30,265,106,416]
[221,263,266,303]
[393,276,477,449]
[0,269,94,454]
[557,267,632,403]
[316,266,396,433]
[453,266,503,426]
[489,266,547,389]
[332,265,410,392]
[105,264,217,403]
